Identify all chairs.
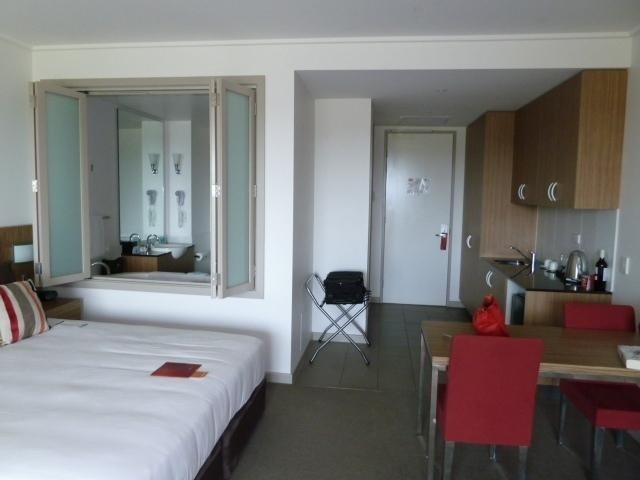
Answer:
[434,333,544,479]
[557,301,640,480]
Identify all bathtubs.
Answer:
[108,272,211,286]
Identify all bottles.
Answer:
[594,248,609,292]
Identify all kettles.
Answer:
[565,249,588,284]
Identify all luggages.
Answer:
[324,271,365,304]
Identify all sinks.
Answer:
[493,261,523,266]
[152,243,191,259]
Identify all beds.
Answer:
[0,317,265,480]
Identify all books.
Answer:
[617,344,640,370]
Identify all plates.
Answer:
[539,265,547,268]
[546,269,558,272]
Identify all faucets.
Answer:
[147,234,159,249]
[91,261,110,274]
[509,245,536,267]
[509,266,535,279]
[130,233,141,246]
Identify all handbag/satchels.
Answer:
[472,295,511,336]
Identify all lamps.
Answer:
[149,153,160,174]
[174,153,182,174]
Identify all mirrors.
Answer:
[117,107,165,244]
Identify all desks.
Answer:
[418,320,640,478]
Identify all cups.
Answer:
[544,259,552,268]
[579,274,589,289]
[544,270,558,281]
[549,261,558,271]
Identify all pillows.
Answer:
[0,278,51,347]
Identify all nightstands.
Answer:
[43,297,81,320]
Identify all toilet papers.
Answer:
[195,250,209,262]
